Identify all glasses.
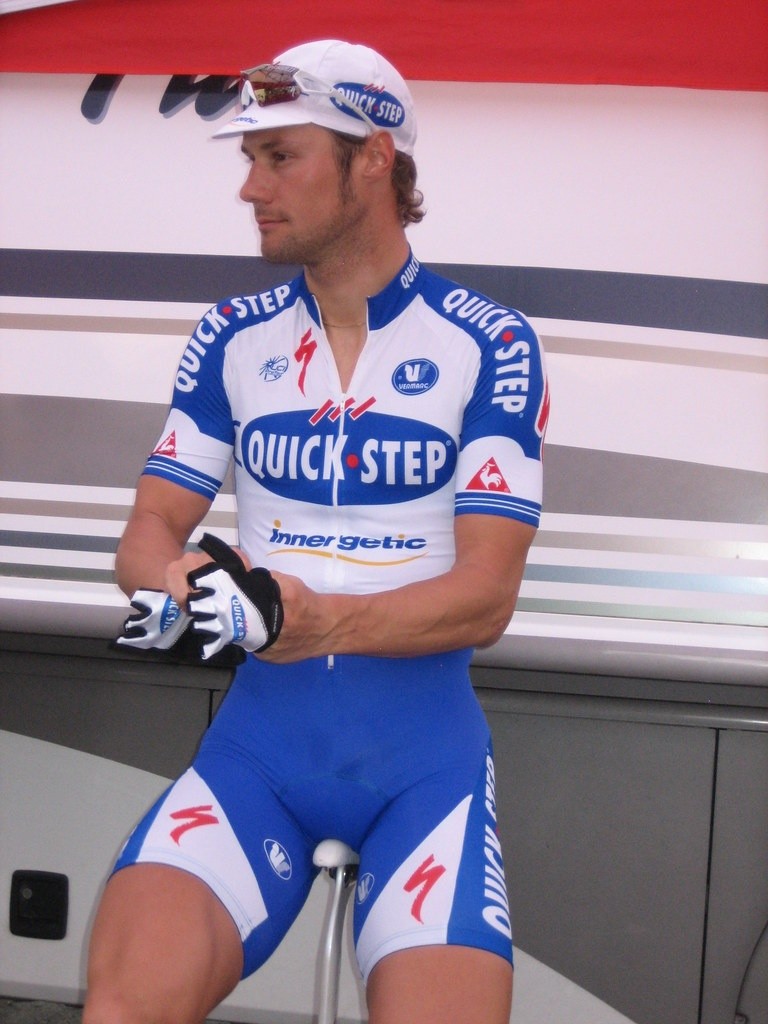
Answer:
[238,61,379,137]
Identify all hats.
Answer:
[211,39,418,156]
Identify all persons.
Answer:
[79,38,549,1023]
